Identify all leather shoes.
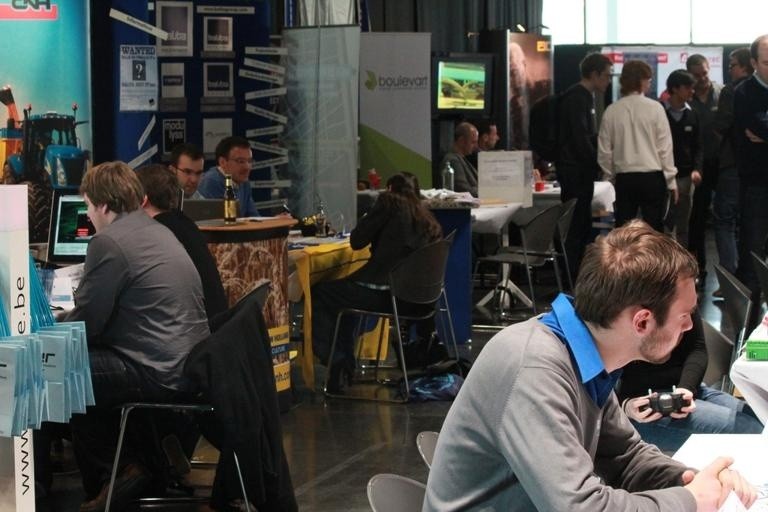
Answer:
[156,435,193,479]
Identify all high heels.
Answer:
[328,358,354,392]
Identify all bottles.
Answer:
[440,161,455,192]
[334,208,345,235]
[315,201,327,238]
[367,166,379,191]
[220,174,238,224]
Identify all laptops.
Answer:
[182,200,225,221]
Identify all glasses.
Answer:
[175,167,204,175]
[230,158,254,164]
[726,64,738,69]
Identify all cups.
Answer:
[534,181,542,193]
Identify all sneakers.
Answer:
[79,463,146,511]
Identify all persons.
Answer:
[554,51,613,289]
[130,164,228,475]
[311,170,445,394]
[597,36,768,300]
[421,217,759,511]
[197,135,258,217]
[441,119,520,247]
[61,162,213,510]
[168,144,204,201]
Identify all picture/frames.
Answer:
[148,0,240,163]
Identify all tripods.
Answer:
[477,222,533,309]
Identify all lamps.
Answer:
[463,24,529,40]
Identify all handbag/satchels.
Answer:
[410,373,464,401]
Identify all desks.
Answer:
[281,230,392,385]
[185,210,302,246]
[366,183,489,340]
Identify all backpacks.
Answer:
[529,84,578,162]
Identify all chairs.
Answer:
[469,204,564,315]
[102,279,276,510]
[708,263,740,338]
[696,319,734,391]
[494,196,577,300]
[366,470,428,512]
[668,191,690,250]
[415,427,442,476]
[323,229,466,404]
